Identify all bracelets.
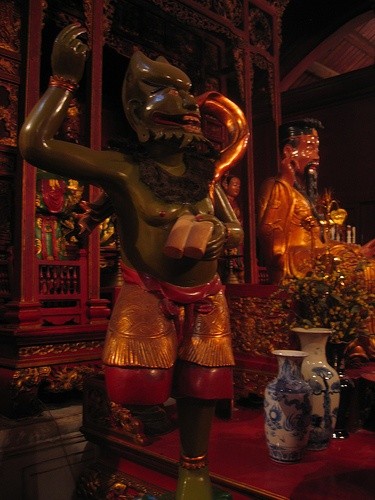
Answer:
[50,79,76,94]
[223,223,229,248]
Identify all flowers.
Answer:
[267,261,375,368]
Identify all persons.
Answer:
[18,22,249,500]
[258,118,375,286]
[222,175,244,282]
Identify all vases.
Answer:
[264,350,313,465]
[292,327,341,450]
[324,343,355,439]
